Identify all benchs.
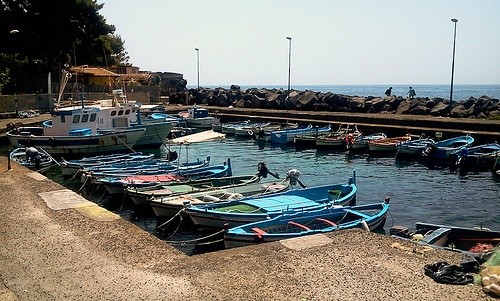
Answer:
[343,209,372,219]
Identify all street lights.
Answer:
[194,48,201,89]
[285,36,292,91]
[10,29,20,117]
[450,18,458,103]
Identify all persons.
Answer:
[385,86,392,96]
[407,87,416,99]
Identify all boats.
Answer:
[315,123,362,149]
[4,44,308,219]
[292,124,336,147]
[220,195,389,249]
[421,135,475,162]
[389,220,500,270]
[451,142,500,170]
[352,132,388,151]
[367,132,420,154]
[270,123,336,146]
[183,168,358,235]
[394,132,444,159]
